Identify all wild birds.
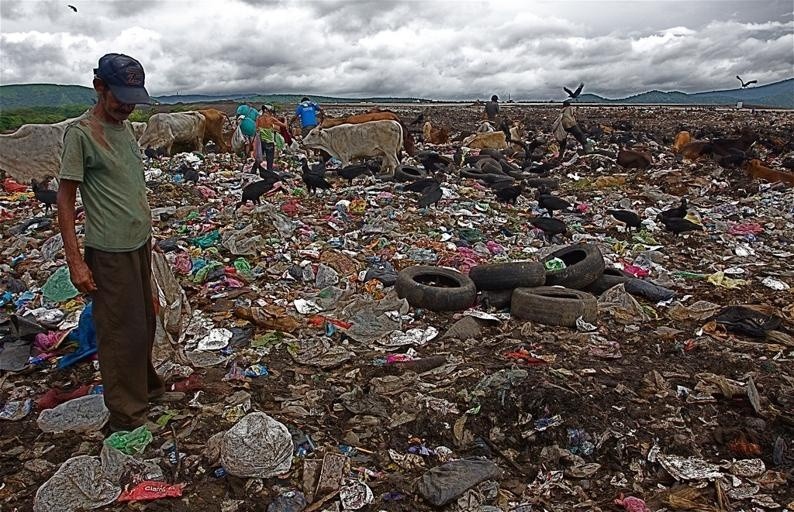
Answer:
[30,175,58,216]
[67,4,79,13]
[563,80,585,98]
[144,144,161,161]
[181,163,206,185]
[604,196,704,239]
[238,150,463,206]
[494,180,574,244]
[736,75,757,87]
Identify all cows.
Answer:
[131,108,233,157]
[301,120,415,174]
[462,111,528,150]
[613,145,650,170]
[318,110,415,160]
[670,122,794,186]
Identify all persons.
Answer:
[556,100,588,160]
[236,101,262,157]
[288,95,326,157]
[479,95,500,122]
[249,103,288,177]
[55,52,187,438]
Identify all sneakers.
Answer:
[111,421,163,434]
[151,390,184,401]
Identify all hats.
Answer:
[95,56,150,104]
